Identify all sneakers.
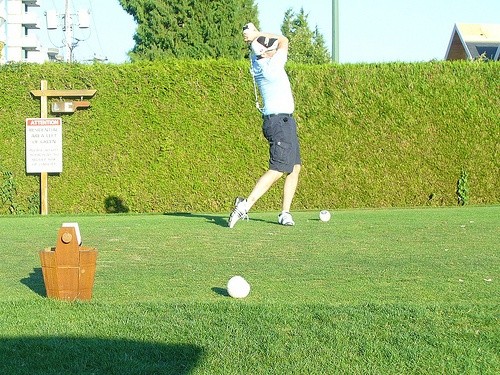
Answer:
[228,196,246,229]
[277,210,294,226]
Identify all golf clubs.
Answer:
[243,25,266,114]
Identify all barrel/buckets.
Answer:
[41,223,98,300]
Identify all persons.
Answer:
[225,23,302,228]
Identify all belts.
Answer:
[262,112,293,119]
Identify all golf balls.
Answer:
[227,275,251,299]
[319,211,330,222]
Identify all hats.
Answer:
[251,35,279,55]
